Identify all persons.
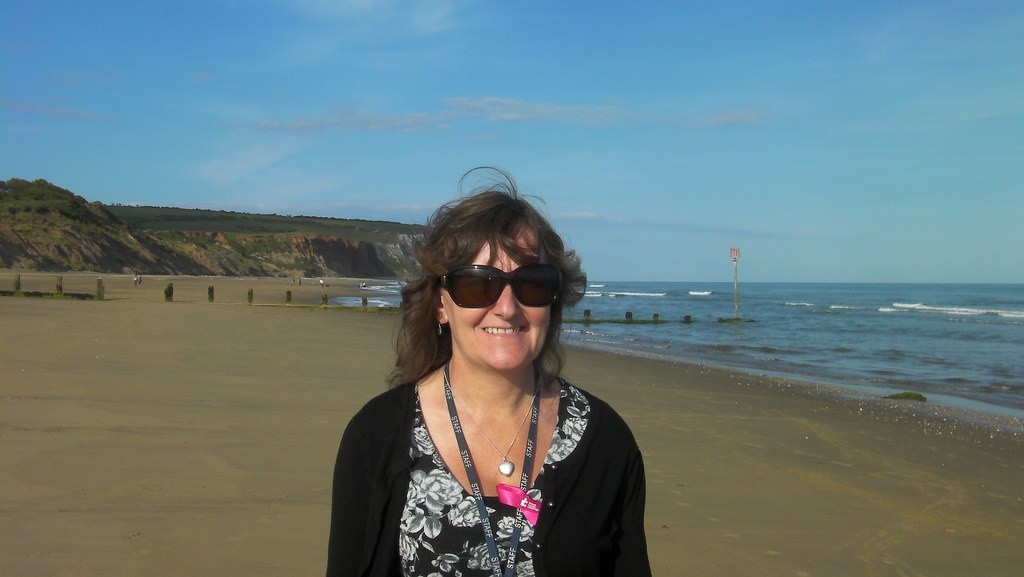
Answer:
[134,274,142,285]
[325,191,653,577]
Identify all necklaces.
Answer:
[441,366,541,477]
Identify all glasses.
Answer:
[439,264,561,308]
